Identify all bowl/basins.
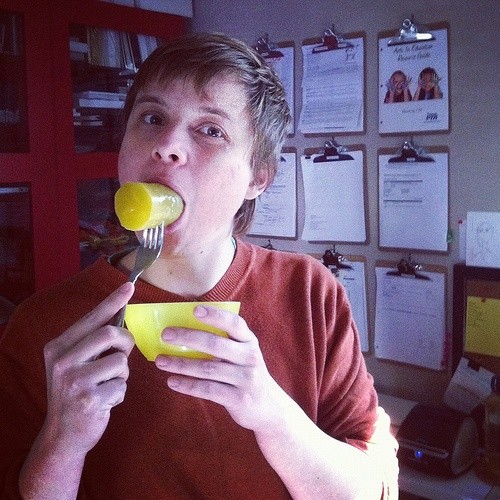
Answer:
[125,302,244,362]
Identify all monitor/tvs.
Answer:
[452,260,500,378]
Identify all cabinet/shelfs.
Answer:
[0,0,187,333]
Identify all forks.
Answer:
[102,221,166,351]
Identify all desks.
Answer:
[378,393,500,500]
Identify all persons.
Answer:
[1,32,401,500]
[384,70,414,103]
[411,68,443,102]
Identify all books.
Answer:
[72,79,134,127]
[71,27,159,71]
